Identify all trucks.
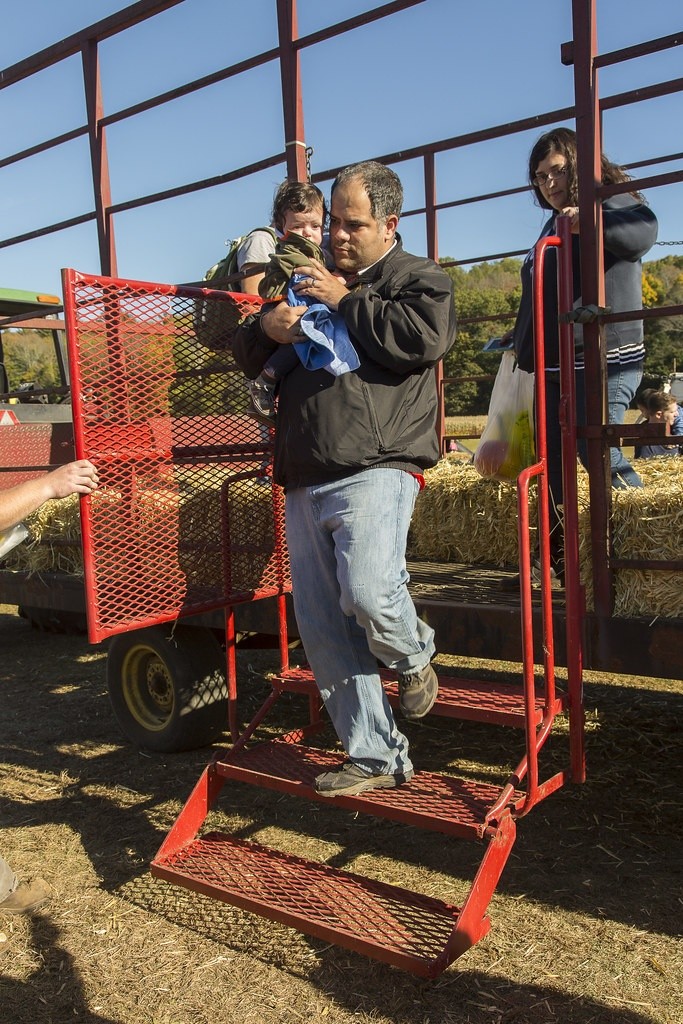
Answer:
[0,0,683,996]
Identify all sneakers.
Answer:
[496,554,565,592]
[312,758,415,798]
[398,663,438,719]
[247,404,277,427]
[249,379,275,417]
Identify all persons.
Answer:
[231,161,456,797]
[475,129,658,591]
[0,460,99,531]
[635,388,683,459]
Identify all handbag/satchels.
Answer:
[473,350,538,486]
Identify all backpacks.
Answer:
[195,258,240,351]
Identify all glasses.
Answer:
[531,166,568,186]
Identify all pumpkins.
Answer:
[475,439,509,477]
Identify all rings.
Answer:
[312,279,315,286]
[299,329,304,336]
